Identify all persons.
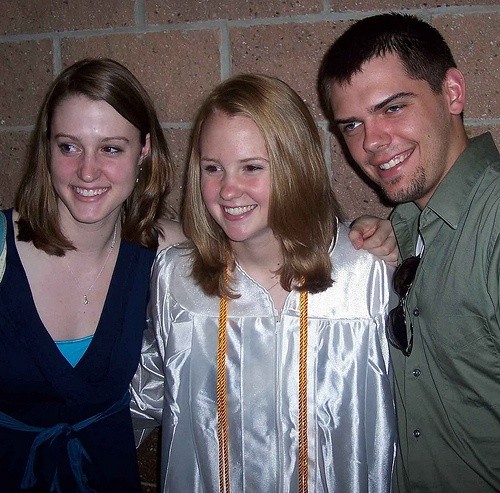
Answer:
[0,58,401,493]
[314,13,500,493]
[128,72,402,493]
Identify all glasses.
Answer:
[385,255,423,357]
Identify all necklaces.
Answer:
[61,218,117,304]
